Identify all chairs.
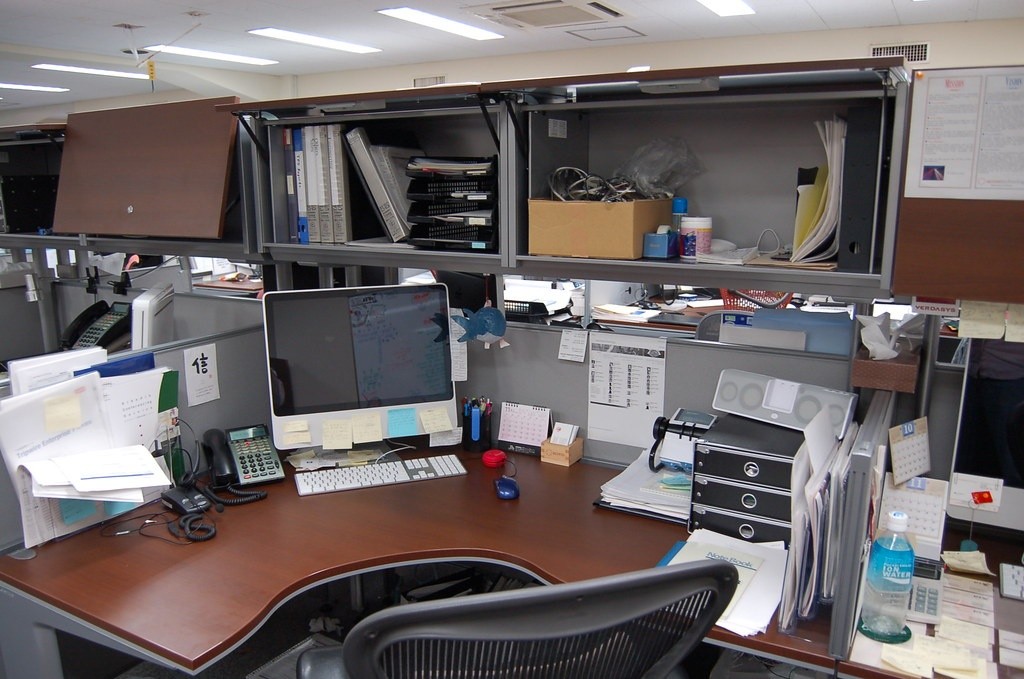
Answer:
[296,561,738,679]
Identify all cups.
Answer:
[680,217,712,258]
[463,414,491,451]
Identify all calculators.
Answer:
[907,552,946,626]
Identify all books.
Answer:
[328,123,386,245]
[281,124,429,248]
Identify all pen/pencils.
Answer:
[462,393,493,418]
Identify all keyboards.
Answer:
[999,563,1024,601]
[294,454,467,496]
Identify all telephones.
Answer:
[56,300,133,352]
[200,422,285,491]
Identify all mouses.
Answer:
[494,474,520,499]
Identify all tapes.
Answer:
[657,224,672,234]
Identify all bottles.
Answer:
[672,197,688,250]
[860,511,914,634]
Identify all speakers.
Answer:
[712,367,859,441]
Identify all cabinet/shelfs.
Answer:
[215,56,909,301]
[0,96,258,263]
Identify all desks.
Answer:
[0,434,835,679]
[835,563,1024,679]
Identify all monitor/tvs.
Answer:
[131,280,176,349]
[262,282,457,466]
[945,337,1024,532]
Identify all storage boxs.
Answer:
[527,197,672,261]
[752,309,852,354]
